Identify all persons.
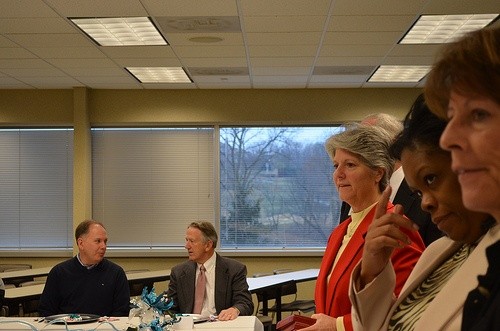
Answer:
[39,219,130,316]
[168,221,254,321]
[298,126,425,331]
[340,113,442,247]
[349,93,500,331]
[425,27,500,331]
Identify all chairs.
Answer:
[254,271,315,331]
[0,264,34,288]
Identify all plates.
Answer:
[165,314,209,322]
[44,314,100,324]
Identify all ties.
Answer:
[192,264,206,314]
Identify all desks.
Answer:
[0,308,265,331]
[0,266,53,290]
[246,269,321,324]
[4,270,172,316]
